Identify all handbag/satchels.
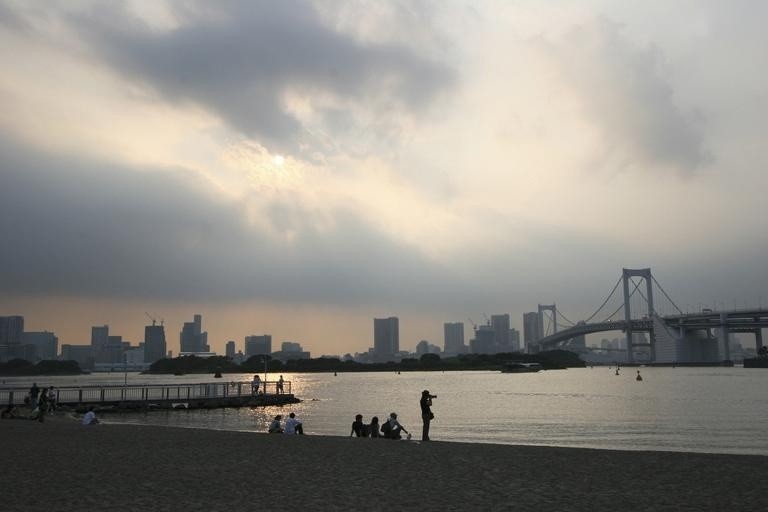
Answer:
[380,420,392,433]
[430,413,433,420]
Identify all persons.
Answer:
[253,374,262,396]
[350,414,371,437]
[81,405,100,425]
[285,413,303,435]
[279,375,284,394]
[269,415,284,433]
[383,412,408,440]
[420,390,434,440]
[0,383,56,424]
[370,417,384,438]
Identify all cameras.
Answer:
[428,395,437,399]
